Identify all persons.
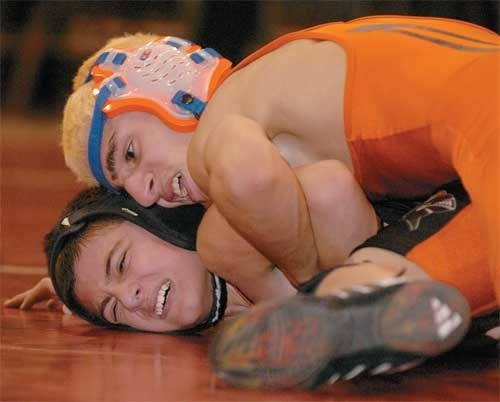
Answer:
[60,15,500,391]
[5,184,471,380]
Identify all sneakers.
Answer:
[209,276,471,390]
